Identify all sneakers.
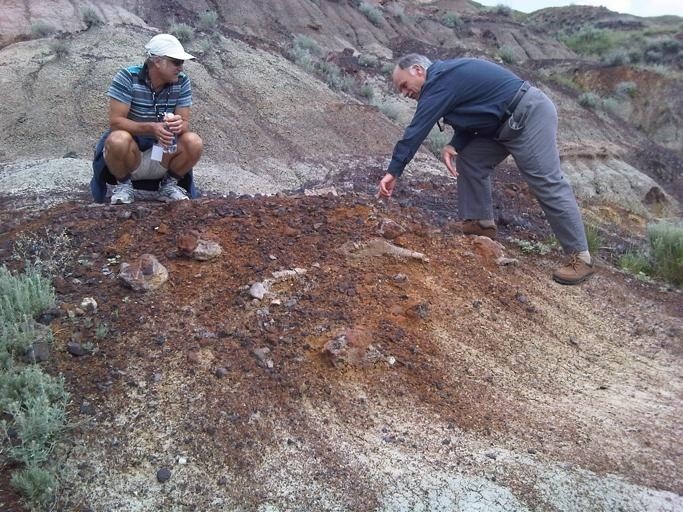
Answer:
[111,184,134,204]
[553,256,594,285]
[155,182,189,203]
[448,219,498,239]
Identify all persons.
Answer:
[375,53,596,285]
[89,34,202,204]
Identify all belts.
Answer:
[493,82,531,134]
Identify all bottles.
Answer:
[160,112,178,154]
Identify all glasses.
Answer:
[162,57,184,65]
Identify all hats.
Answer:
[145,34,197,62]
